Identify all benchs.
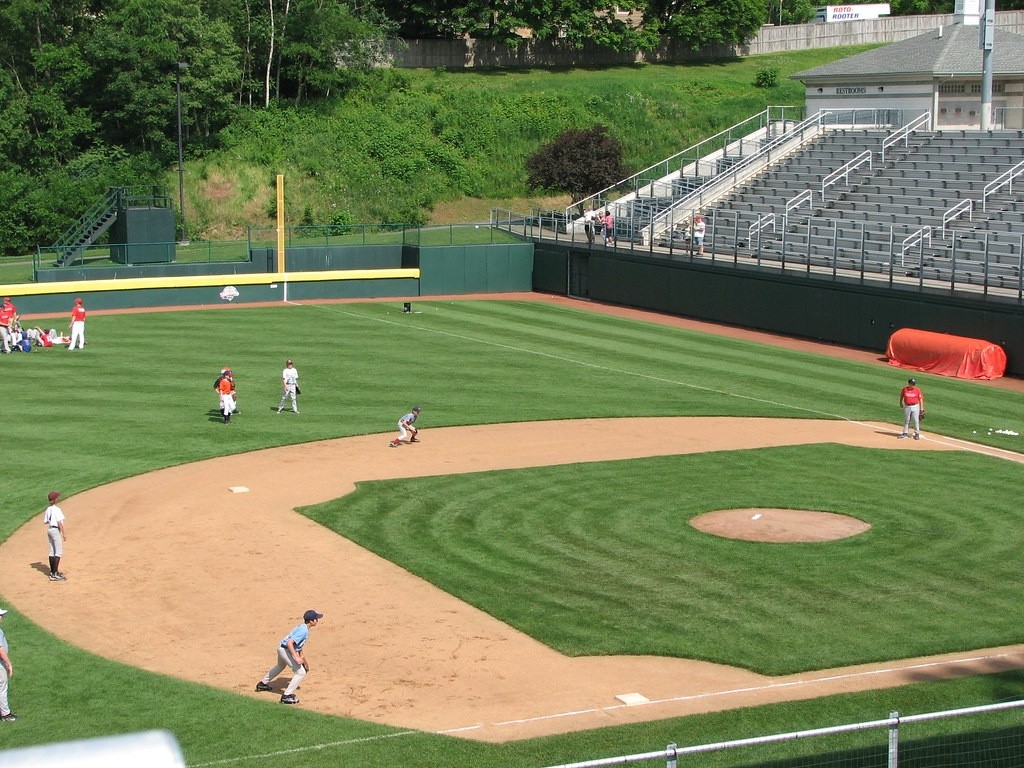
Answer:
[957,227,1024,241]
[973,219,1024,232]
[942,153,1024,164]
[988,209,1024,221]
[1003,201,1024,211]
[942,145,1024,155]
[942,179,1009,193]
[942,207,971,218]
[924,256,1024,276]
[942,170,1023,183]
[950,238,1024,254]
[942,137,1024,147]
[941,198,980,209]
[942,161,1024,173]
[1014,192,1024,202]
[916,267,1024,290]
[942,130,1024,138]
[941,188,991,199]
[654,128,941,273]
[932,246,1024,264]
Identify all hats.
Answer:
[908,379,916,386]
[3,297,10,301]
[0,609,8,615]
[49,492,61,501]
[303,610,323,619]
[694,216,701,222]
[0,303,7,307]
[75,298,82,304]
[286,360,293,365]
[413,406,420,411]
[220,367,233,377]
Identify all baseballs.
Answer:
[972,428,1018,436]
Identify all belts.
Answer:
[281,645,287,648]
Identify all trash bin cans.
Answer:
[403,302,412,312]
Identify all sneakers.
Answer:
[281,695,299,704]
[256,682,272,691]
[2,712,20,722]
[49,572,66,581]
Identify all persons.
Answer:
[585,211,615,245]
[214,369,242,423]
[255,610,323,703]
[44,492,67,581]
[0,297,86,353]
[695,216,705,256]
[682,218,692,256]
[0,608,16,723]
[389,406,420,447]
[897,379,923,439]
[276,360,301,413]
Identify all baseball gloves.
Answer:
[300,657,310,673]
[411,428,417,435]
[231,391,237,401]
[918,409,926,422]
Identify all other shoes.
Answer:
[915,434,919,440]
[411,438,419,442]
[695,253,703,257]
[295,412,299,413]
[276,411,281,414]
[899,433,908,438]
[389,443,397,447]
